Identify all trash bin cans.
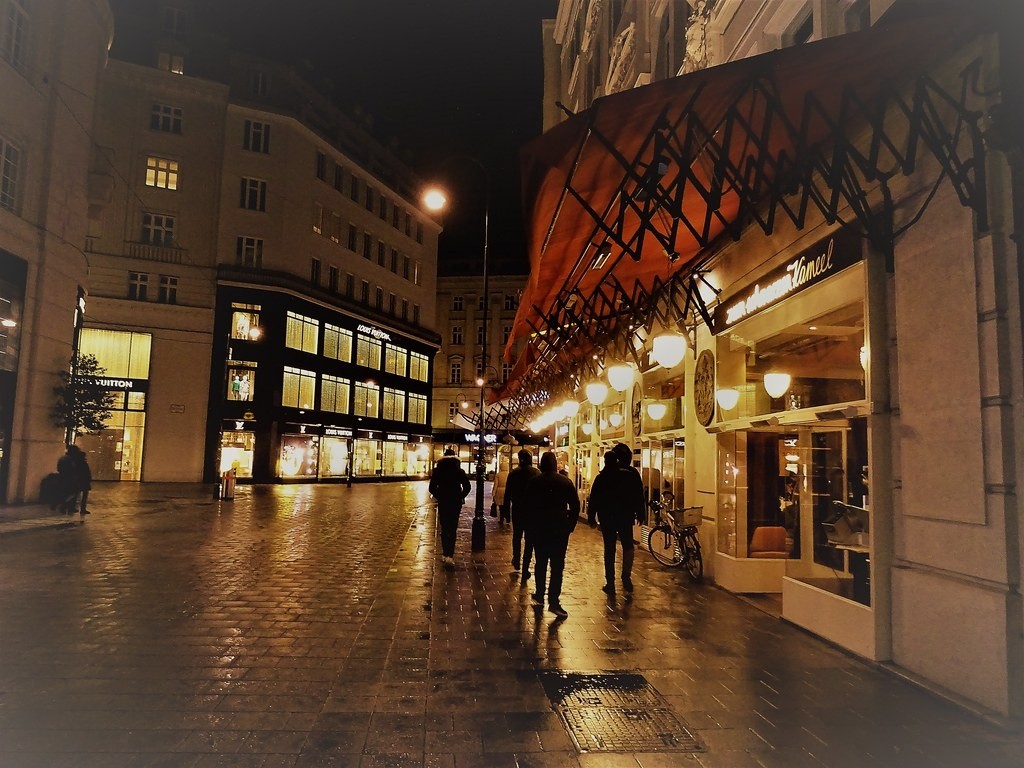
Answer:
[220,468,236,502]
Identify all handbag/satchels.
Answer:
[489,498,498,518]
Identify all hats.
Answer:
[541,453,556,467]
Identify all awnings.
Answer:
[485,5,998,406]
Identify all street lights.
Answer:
[423,152,495,553]
[452,392,469,452]
[476,364,499,476]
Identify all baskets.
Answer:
[674,505,703,528]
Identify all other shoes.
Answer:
[506,523,511,531]
[548,603,568,618]
[511,558,521,570]
[602,583,616,596]
[622,576,634,593]
[522,570,532,580]
[441,553,455,567]
[531,594,545,606]
[80,505,91,514]
[66,505,80,513]
[497,520,504,525]
[59,506,66,514]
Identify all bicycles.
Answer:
[646,490,704,582]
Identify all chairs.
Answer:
[750,527,791,559]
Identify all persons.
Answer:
[57,445,92,515]
[231,375,250,401]
[429,448,471,567]
[492,443,647,617]
